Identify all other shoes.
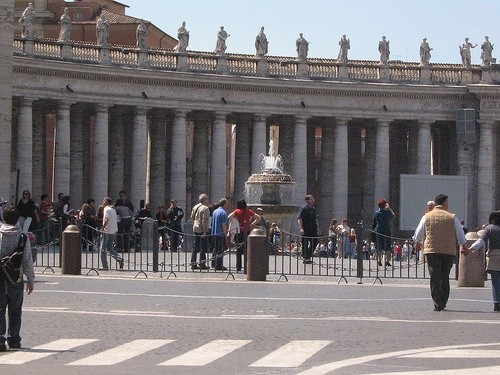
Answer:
[434,307,444,311]
[378,262,382,266]
[494,303,500,311]
[0,343,6,351]
[303,258,315,264]
[191,265,199,269]
[215,266,227,270]
[9,343,21,348]
[119,258,124,269]
[199,264,210,269]
[385,262,392,266]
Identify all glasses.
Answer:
[23,193,29,195]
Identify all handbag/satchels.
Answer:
[0,233,28,283]
[485,249,500,273]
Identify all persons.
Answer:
[288,218,375,259]
[462,210,500,311]
[80,190,134,268]
[0,206,35,352]
[371,200,394,265]
[96,14,110,45]
[191,194,210,269]
[17,190,72,244]
[135,200,184,251]
[210,197,227,270]
[419,38,433,67]
[255,207,281,250]
[135,19,390,64]
[412,194,469,310]
[297,194,320,264]
[459,36,494,66]
[228,199,260,270]
[393,240,420,260]
[57,8,72,42]
[19,2,35,38]
